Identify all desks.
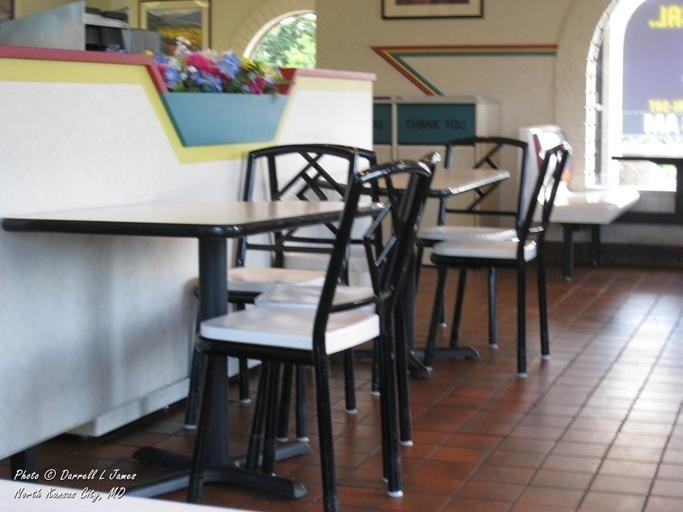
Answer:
[1,197,386,502]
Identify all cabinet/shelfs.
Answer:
[372,94,500,270]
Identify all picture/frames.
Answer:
[137,0,212,56]
[378,1,483,20]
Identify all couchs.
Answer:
[518,123,642,280]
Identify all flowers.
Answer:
[145,34,296,96]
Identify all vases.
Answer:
[162,91,289,148]
